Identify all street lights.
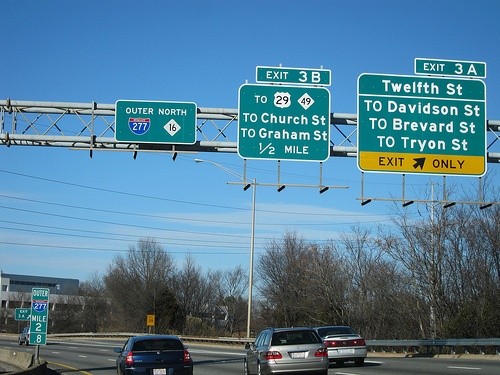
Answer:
[194,158,256,338]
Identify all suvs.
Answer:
[244,327,330,375]
[18,327,31,346]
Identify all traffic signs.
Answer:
[356,74,489,178]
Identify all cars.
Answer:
[114,334,193,375]
[312,326,369,365]
